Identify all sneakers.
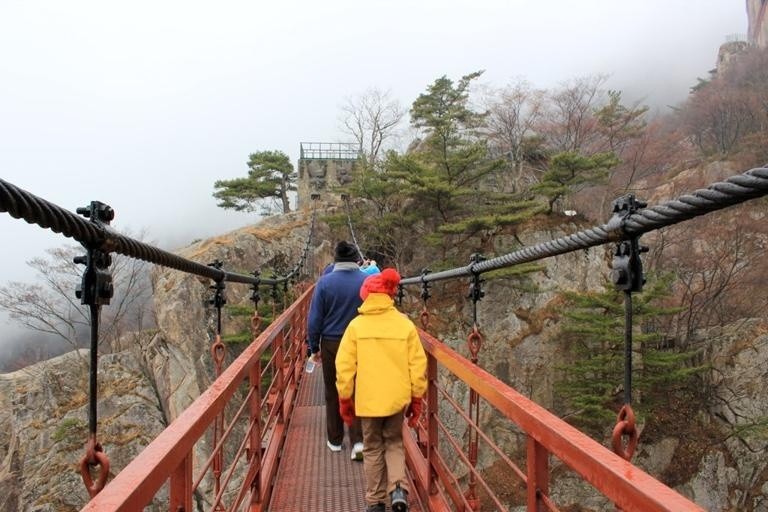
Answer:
[327,441,342,452]
[390,480,408,512]
[365,501,386,512]
[350,442,363,461]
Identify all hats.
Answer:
[333,240,360,262]
[359,267,400,301]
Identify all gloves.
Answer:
[339,397,355,429]
[405,396,421,428]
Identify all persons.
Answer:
[307,239,368,461]
[335,269,429,512]
[324,257,381,276]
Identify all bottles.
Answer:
[305,351,316,373]
